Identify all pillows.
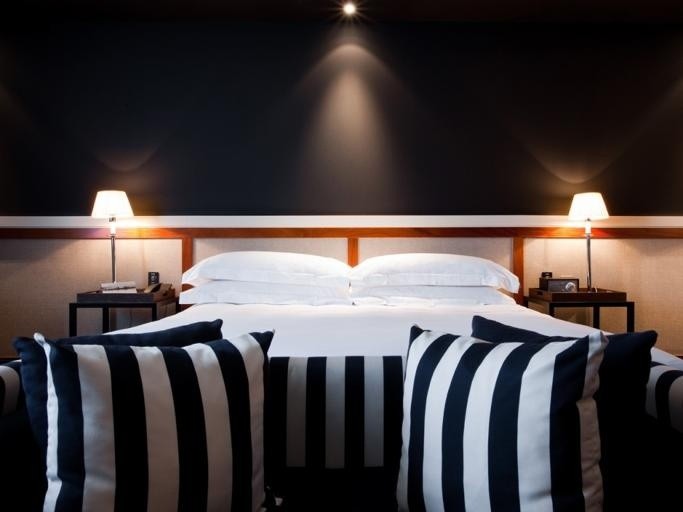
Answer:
[12,319,224,512]
[33,330,277,512]
[348,285,516,308]
[178,281,353,305]
[394,323,609,512]
[180,251,352,288]
[348,252,520,294]
[469,314,658,512]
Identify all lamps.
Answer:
[568,192,610,294]
[90,189,134,294]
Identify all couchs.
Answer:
[0,352,683,512]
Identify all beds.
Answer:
[103,251,683,370]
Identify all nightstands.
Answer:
[523,294,635,335]
[67,295,180,335]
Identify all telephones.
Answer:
[144,282,172,295]
